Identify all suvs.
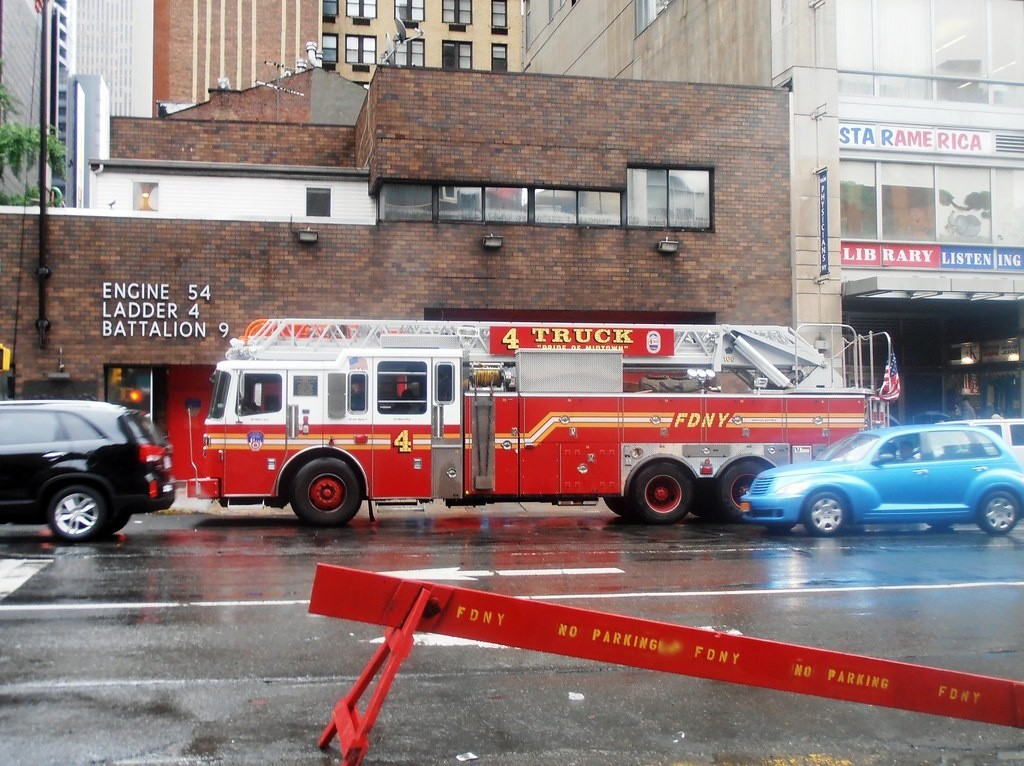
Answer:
[1,401,176,543]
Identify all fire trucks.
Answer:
[186,317,889,527]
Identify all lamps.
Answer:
[288,213,319,242]
[657,241,680,252]
[482,235,503,248]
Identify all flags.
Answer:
[880,347,900,402]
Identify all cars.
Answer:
[739,423,1024,535]
